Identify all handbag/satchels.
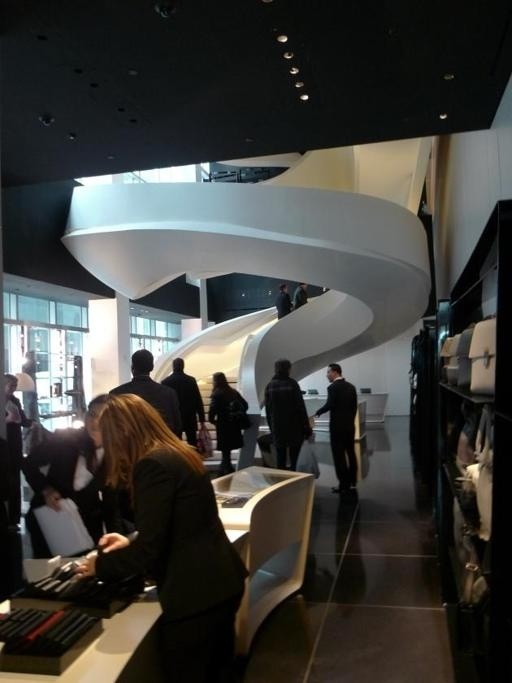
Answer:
[440,316,498,396]
[457,405,496,544]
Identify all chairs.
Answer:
[169,398,368,472]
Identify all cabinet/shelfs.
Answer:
[411,200,512,682]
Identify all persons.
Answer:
[263,356,315,471]
[68,392,250,683]
[313,361,359,494]
[291,278,307,310]
[159,353,206,457]
[274,282,293,320]
[208,371,249,477]
[18,392,132,555]
[0,373,39,531]
[108,349,184,443]
[21,350,40,430]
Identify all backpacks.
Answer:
[224,388,252,430]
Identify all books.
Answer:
[32,497,94,564]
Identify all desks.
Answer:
[1,465,318,683]
